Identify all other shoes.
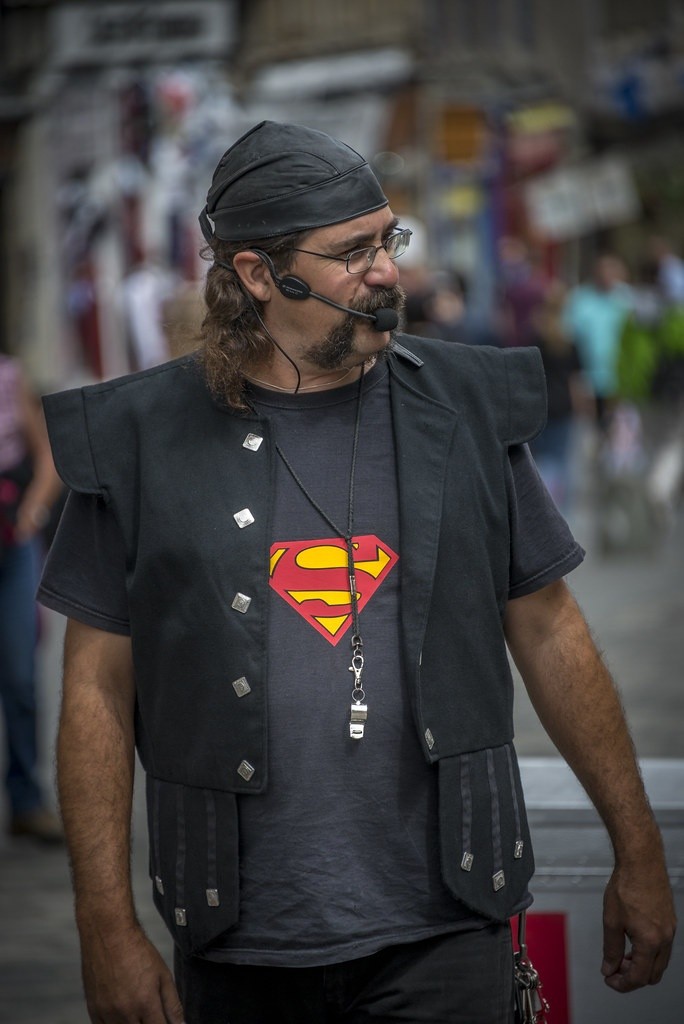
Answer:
[7,806,70,846]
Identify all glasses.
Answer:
[278,227,412,273]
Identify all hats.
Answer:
[199,119,388,241]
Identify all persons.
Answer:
[118,223,684,558]
[37,120,675,1024]
[0,352,69,847]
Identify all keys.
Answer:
[514,960,550,1024]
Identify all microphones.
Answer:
[278,273,401,332]
